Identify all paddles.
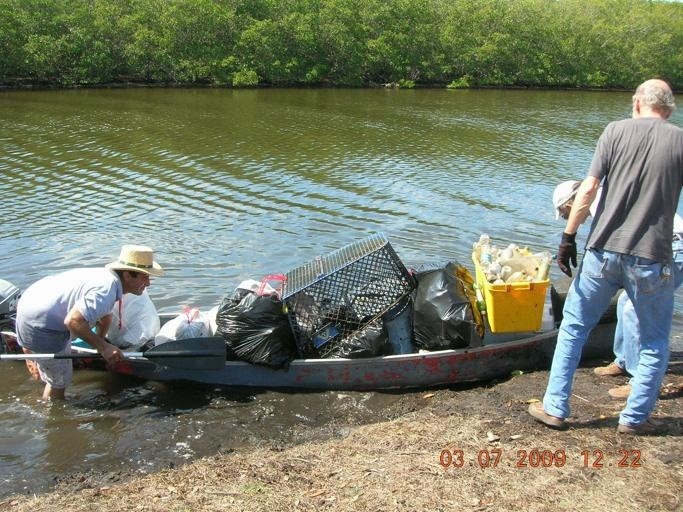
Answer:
[0,337,226,371]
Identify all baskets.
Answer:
[281,233,414,358]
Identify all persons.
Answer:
[14,242,163,398]
[550,180,682,399]
[526,79,683,432]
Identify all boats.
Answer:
[0,242,683,392]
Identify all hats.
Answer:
[105,244,163,277]
[552,180,576,219]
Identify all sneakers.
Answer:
[595,363,623,376]
[609,385,631,398]
[617,418,661,434]
[528,401,564,428]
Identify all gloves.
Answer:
[557,231,578,276]
